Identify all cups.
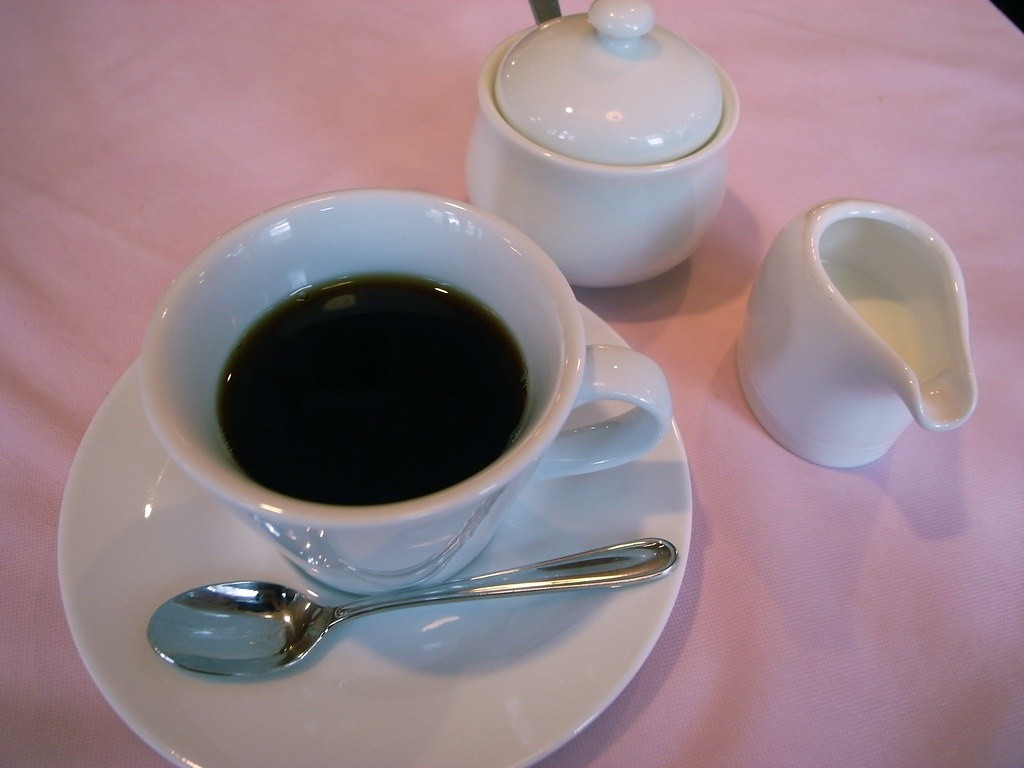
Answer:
[138,187,673,596]
[464,0,740,288]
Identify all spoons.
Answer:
[146,537,679,677]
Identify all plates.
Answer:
[56,299,691,768]
